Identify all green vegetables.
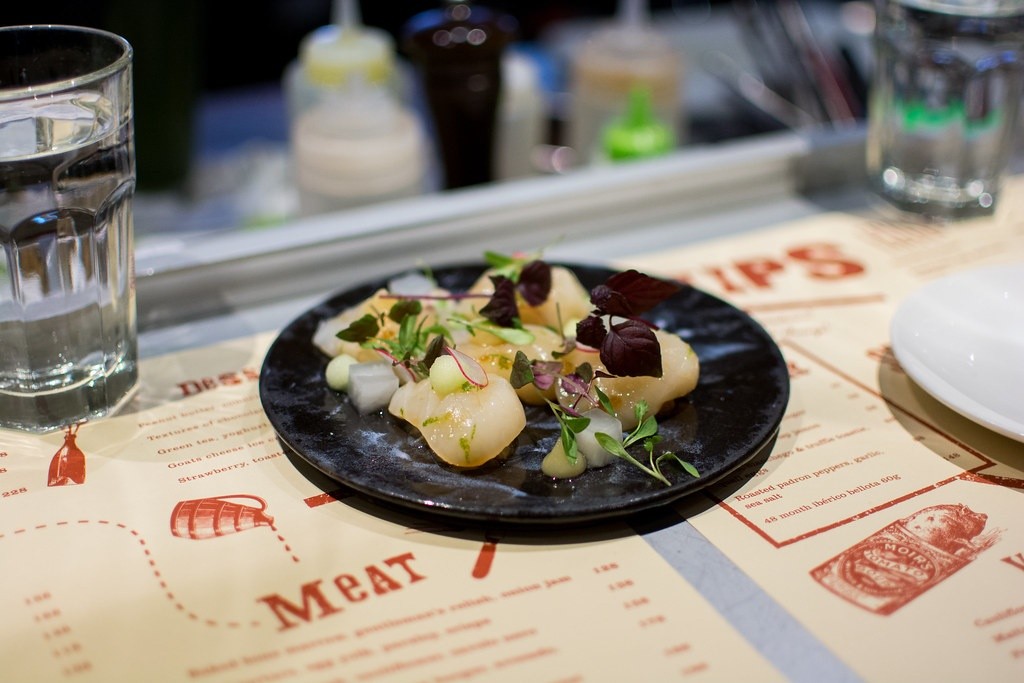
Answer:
[336,251,701,487]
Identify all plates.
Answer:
[890,258,1024,444]
[259,262,789,523]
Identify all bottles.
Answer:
[599,88,673,162]
[573,0,680,164]
[285,1,434,217]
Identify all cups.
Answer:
[0,25,141,435]
[863,0,1022,218]
